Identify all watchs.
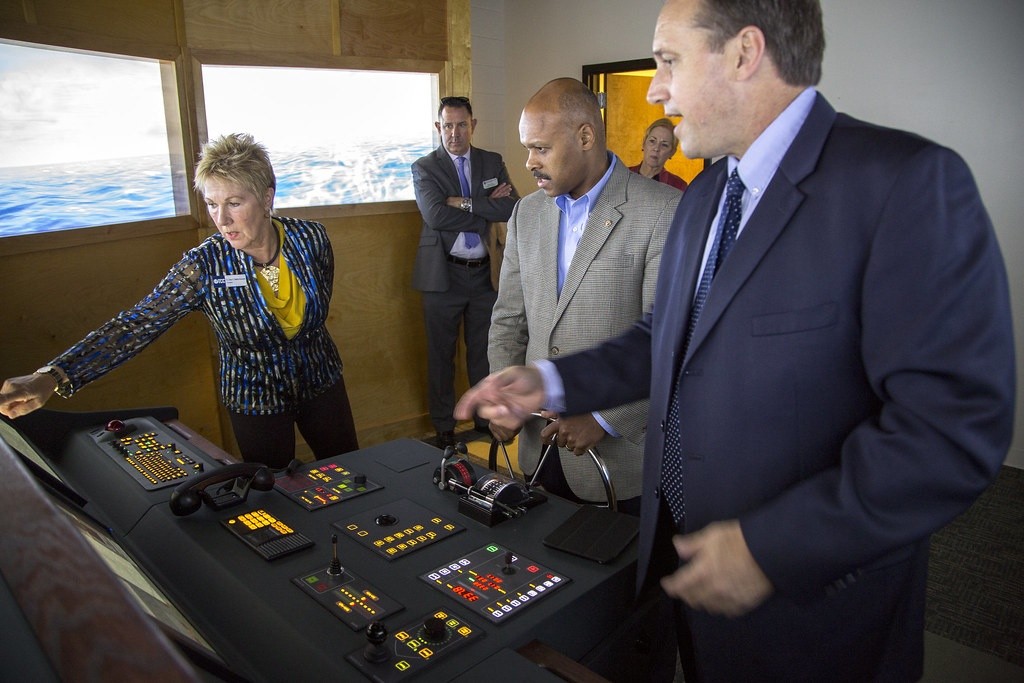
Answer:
[459,195,472,213]
[31,367,63,391]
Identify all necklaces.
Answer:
[251,222,280,292]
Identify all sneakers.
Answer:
[475,423,510,443]
[437,430,455,451]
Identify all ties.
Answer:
[457,157,480,249]
[660,167,745,525]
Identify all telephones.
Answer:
[168,462,275,516]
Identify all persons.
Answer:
[486,75,688,514]
[624,118,687,193]
[2,135,359,471]
[411,98,522,450]
[450,1,1014,683]
